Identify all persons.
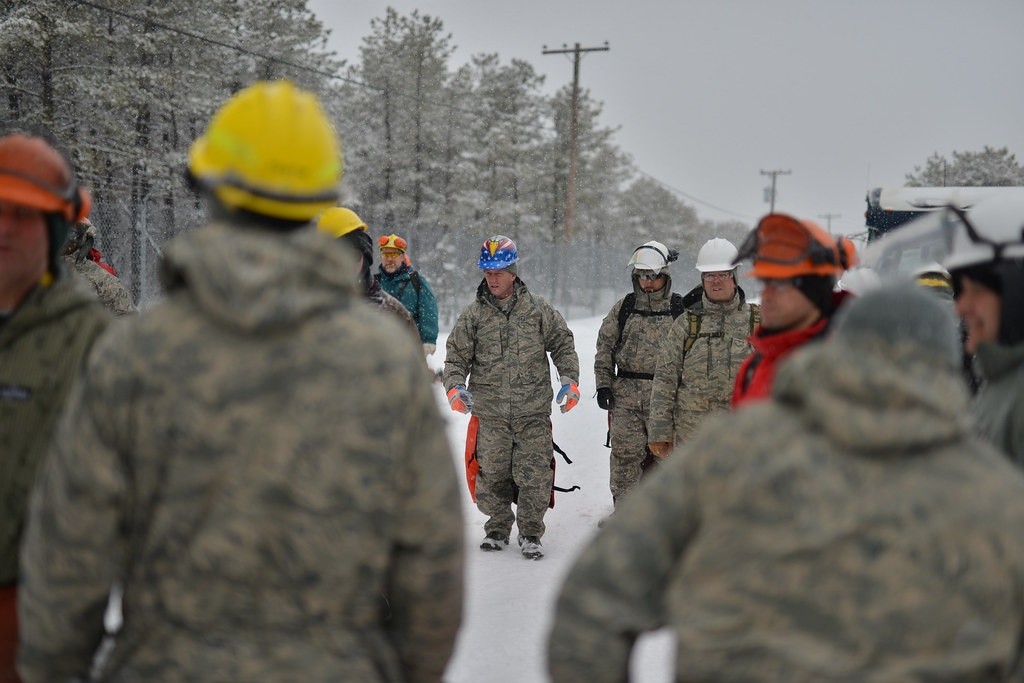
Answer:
[942,198,1024,466]
[57,216,140,320]
[549,278,1024,683]
[317,207,422,346]
[594,241,685,528]
[729,214,855,411]
[911,261,982,399]
[0,136,118,683]
[374,234,440,357]
[443,235,581,560]
[16,80,465,683]
[648,238,762,461]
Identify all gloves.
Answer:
[596,388,614,411]
[556,376,580,414]
[445,385,472,414]
[423,343,436,355]
[648,441,673,457]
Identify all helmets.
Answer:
[633,241,673,273]
[376,234,407,254]
[313,207,367,241]
[750,214,856,279]
[696,238,743,273]
[912,261,950,279]
[942,186,1024,267]
[834,261,882,301]
[477,235,519,269]
[184,80,346,221]
[0,135,91,221]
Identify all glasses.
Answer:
[633,270,661,280]
[700,272,733,282]
[379,252,400,260]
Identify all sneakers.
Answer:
[517,534,545,561]
[479,531,511,552]
